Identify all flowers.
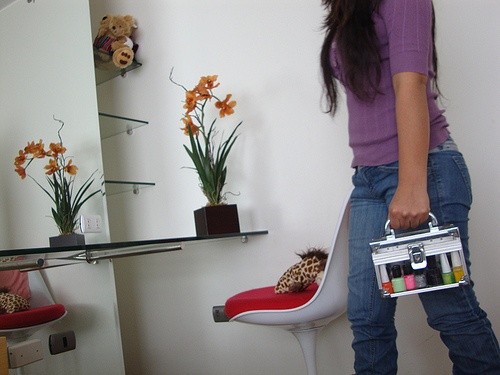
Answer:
[14,114,101,234]
[169,66,243,204]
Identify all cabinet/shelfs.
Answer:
[94,47,155,194]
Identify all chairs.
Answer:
[0,270,68,375]
[225,185,357,375]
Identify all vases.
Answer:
[194,205,240,236]
[50,234,85,247]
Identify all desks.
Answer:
[0,231,268,272]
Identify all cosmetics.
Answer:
[379,248,464,293]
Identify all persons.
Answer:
[319,0,500,375]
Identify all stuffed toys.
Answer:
[94,15,138,69]
